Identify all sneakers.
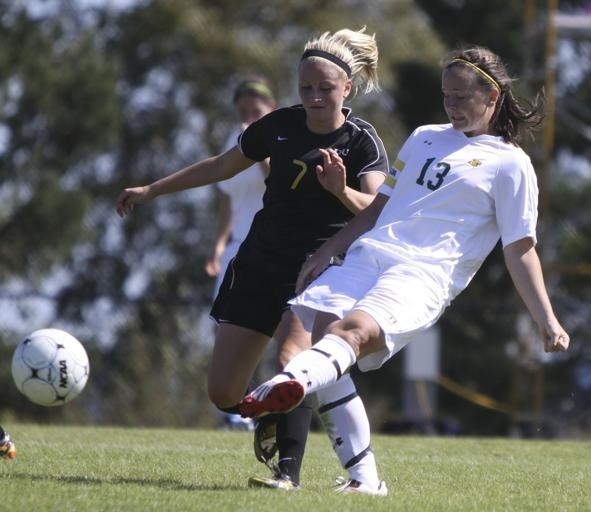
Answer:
[237,378,308,421]
[248,474,301,491]
[332,478,388,497]
[253,414,279,464]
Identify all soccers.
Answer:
[11,328,90,408]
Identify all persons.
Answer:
[114,25,390,498]
[201,74,282,431]
[237,47,571,496]
